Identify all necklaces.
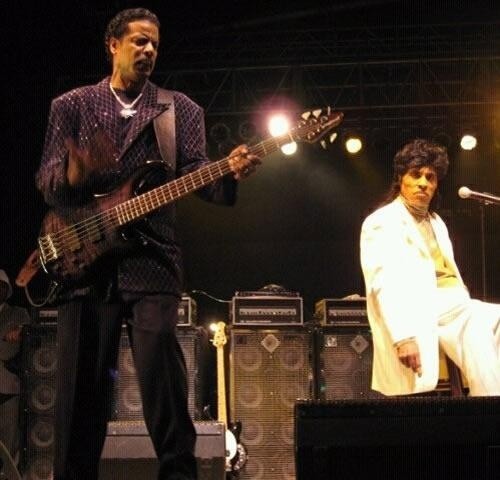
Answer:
[108,83,144,119]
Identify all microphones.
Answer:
[458,186,500,206]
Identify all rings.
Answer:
[243,167,252,174]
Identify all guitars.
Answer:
[37,107,344,290]
[203,321,248,472]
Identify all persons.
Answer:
[35,8,263,480]
[0,268,33,480]
[358,136,500,397]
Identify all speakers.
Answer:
[21,324,77,479]
[313,329,373,400]
[115,329,218,420]
[224,329,309,480]
